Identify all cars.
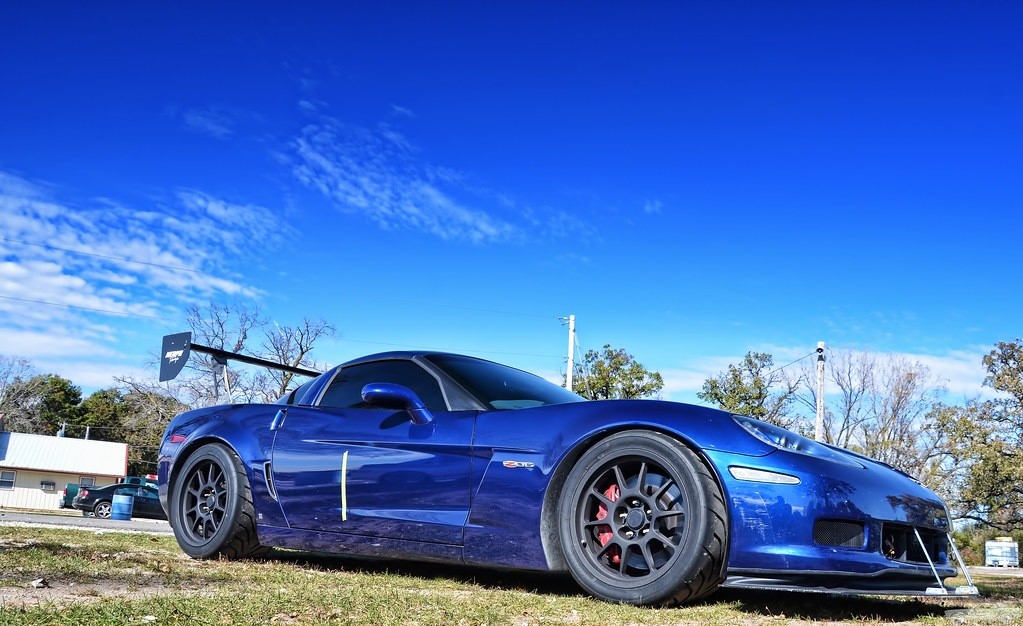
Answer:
[72,484,167,520]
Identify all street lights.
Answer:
[814,342,826,442]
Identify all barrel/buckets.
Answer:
[110,495,134,520]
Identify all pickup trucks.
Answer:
[58,477,159,518]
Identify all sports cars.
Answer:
[155,331,958,607]
